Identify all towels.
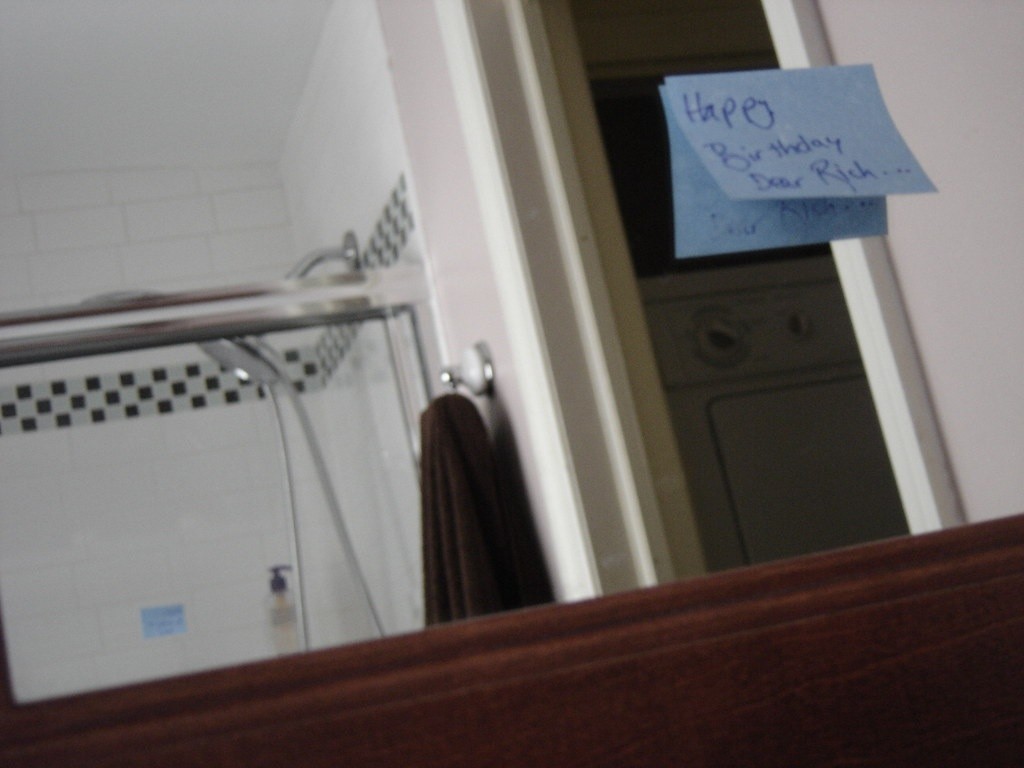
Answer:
[415,391,534,626]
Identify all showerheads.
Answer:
[86,285,291,386]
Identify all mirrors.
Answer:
[0,0,1024,709]
